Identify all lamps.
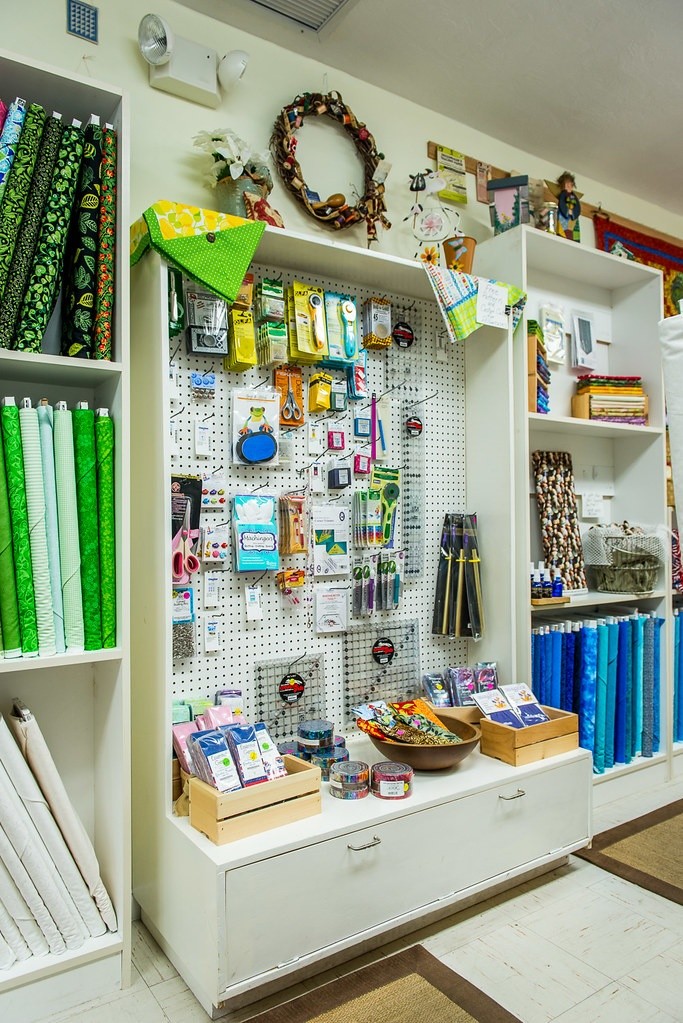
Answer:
[138,12,251,111]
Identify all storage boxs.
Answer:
[186,754,321,844]
[479,706,579,767]
[420,694,482,726]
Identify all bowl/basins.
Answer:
[368,712,483,770]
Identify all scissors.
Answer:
[172,499,201,579]
[281,373,302,421]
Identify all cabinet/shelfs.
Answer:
[474,221,683,839]
[0,47,134,1023]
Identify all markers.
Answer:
[393,567,400,610]
[368,567,375,616]
